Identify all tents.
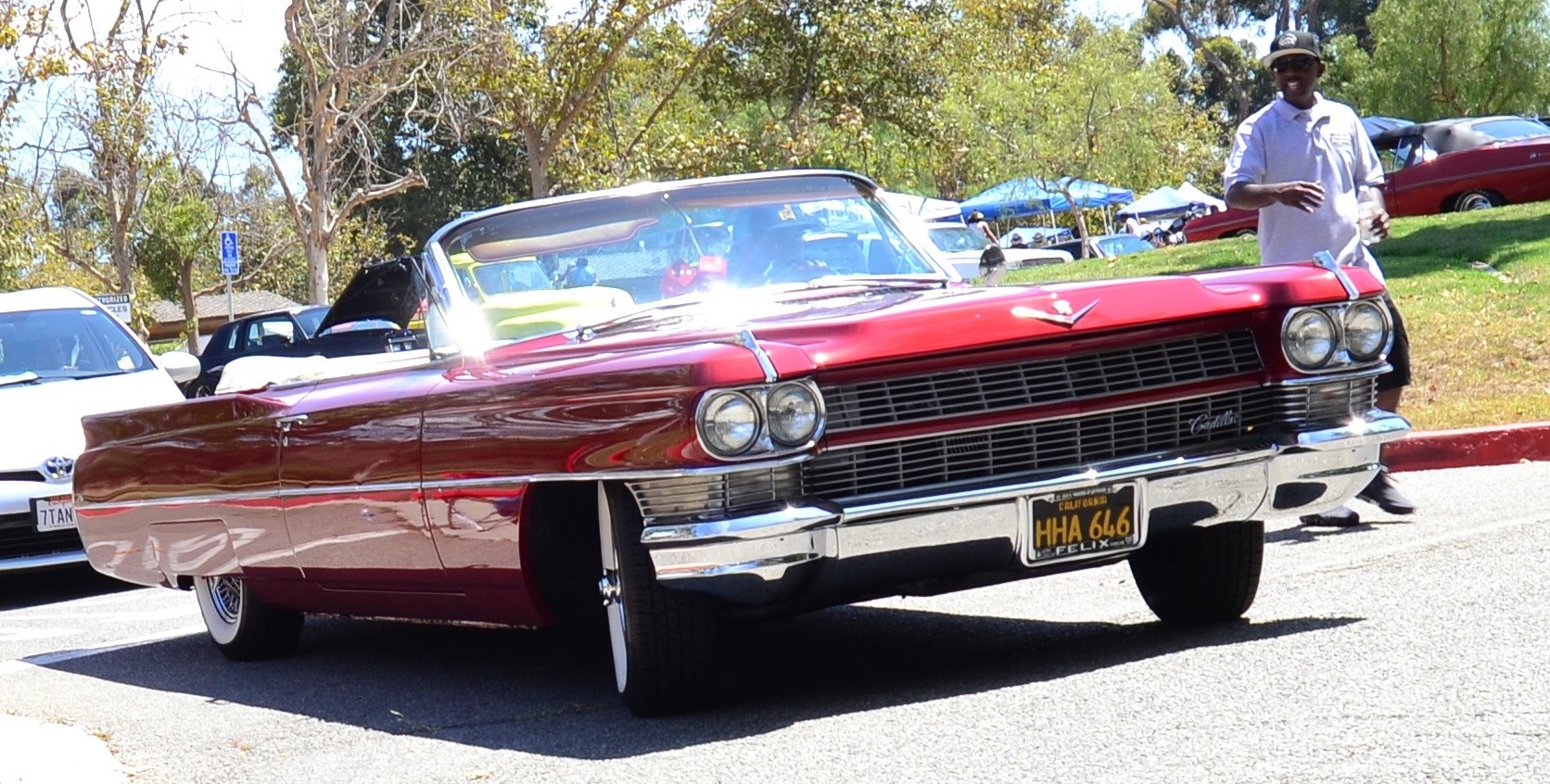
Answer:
[958,176,1141,244]
[1115,181,1226,228]
[875,188,964,224]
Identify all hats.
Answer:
[1198,203,1205,209]
[1033,233,1043,242]
[1140,217,1147,222]
[1263,29,1322,73]
[1121,212,1129,217]
[1211,205,1219,210]
[1010,233,1023,245]
[576,258,587,266]
[968,209,984,219]
[1072,226,1079,236]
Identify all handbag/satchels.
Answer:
[1149,227,1167,248]
[1165,231,1177,243]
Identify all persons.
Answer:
[1226,31,1413,526]
[1118,212,1152,239]
[1031,232,1046,249]
[1210,204,1221,214]
[968,208,998,245]
[569,257,594,287]
[1525,105,1538,121]
[1009,233,1028,248]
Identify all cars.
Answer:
[0,286,202,587]
[1041,233,1155,263]
[186,255,434,404]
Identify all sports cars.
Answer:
[1181,114,1550,244]
[66,170,1413,725]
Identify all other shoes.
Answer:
[1355,468,1414,513]
[1299,506,1360,528]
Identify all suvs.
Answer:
[921,220,1075,281]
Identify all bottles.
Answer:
[1357,185,1381,246]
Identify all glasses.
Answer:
[1270,54,1319,74]
